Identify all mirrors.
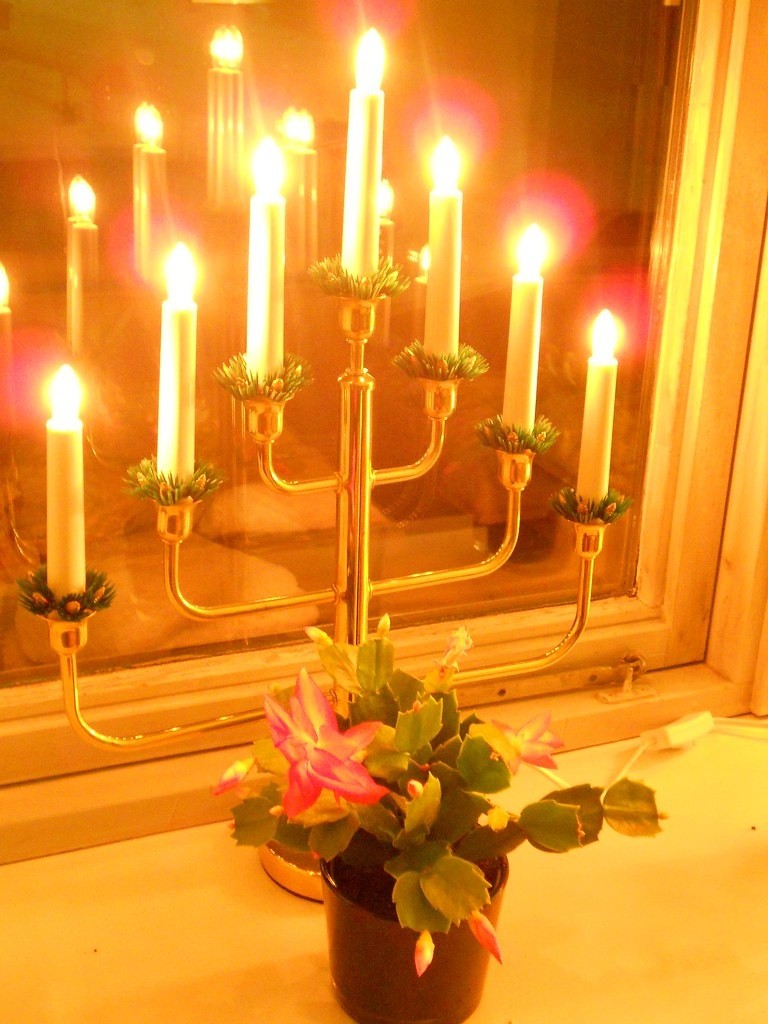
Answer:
[1,0,767,787]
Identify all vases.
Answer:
[317,859,510,1024]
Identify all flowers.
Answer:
[206,615,570,981]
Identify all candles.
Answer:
[245,192,295,385]
[342,90,385,280]
[500,275,548,440]
[158,296,202,482]
[43,415,87,599]
[422,190,461,373]
[578,356,617,509]
